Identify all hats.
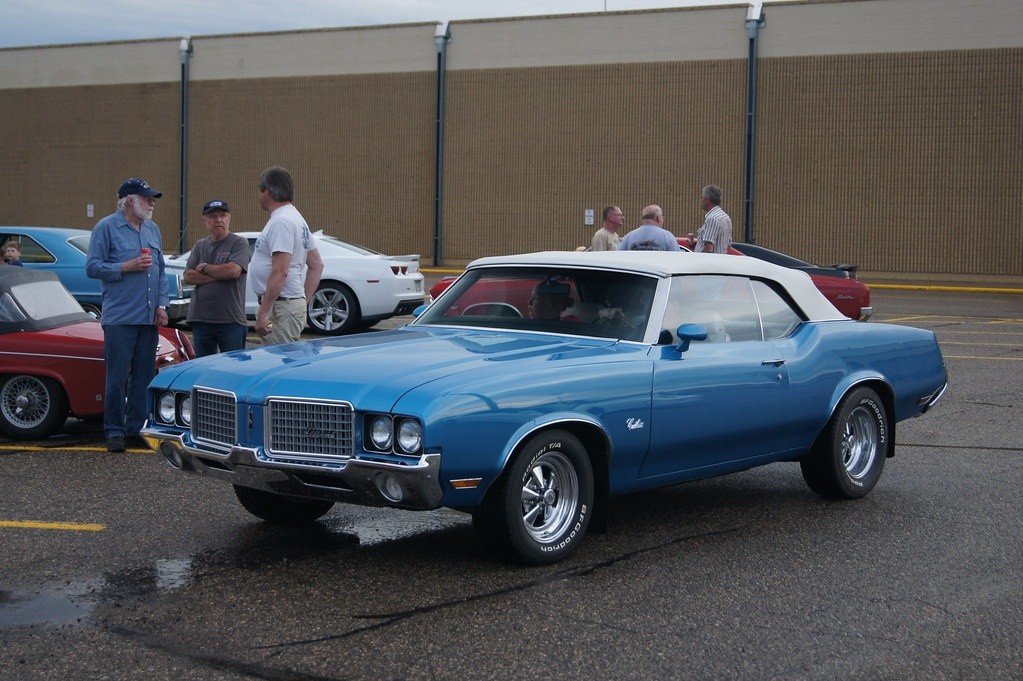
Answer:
[203,200,230,215]
[118,178,162,198]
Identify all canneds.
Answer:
[140,248,151,256]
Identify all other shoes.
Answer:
[125,436,149,449]
[107,437,126,452]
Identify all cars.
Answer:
[0,269,199,442]
[0,226,194,323]
[431,241,872,348]
[154,231,426,331]
[140,250,949,565]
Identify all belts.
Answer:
[258,295,304,302]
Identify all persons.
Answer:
[250,166,324,347]
[183,198,250,358]
[5,241,23,267]
[592,205,625,251]
[617,205,680,251]
[687,185,732,254]
[86,178,169,452]
[528,281,568,319]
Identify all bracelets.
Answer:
[158,305,166,310]
[201,263,208,275]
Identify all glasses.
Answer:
[138,197,159,204]
[206,214,230,221]
